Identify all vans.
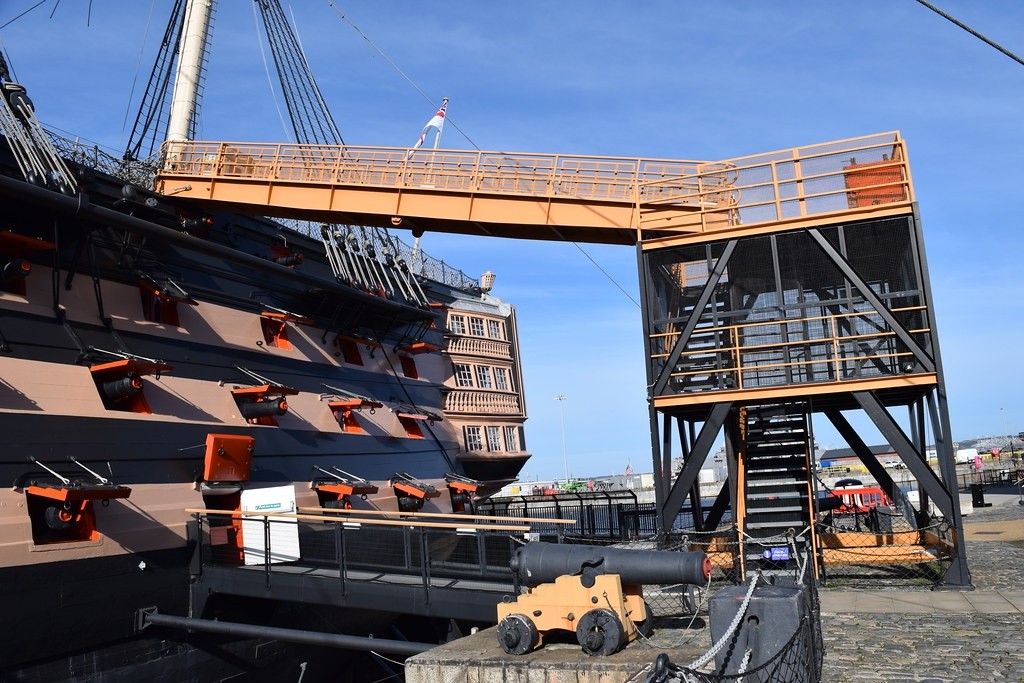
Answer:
[885,461,900,467]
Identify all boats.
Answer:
[0,0,529,683]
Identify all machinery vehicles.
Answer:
[496,543,714,654]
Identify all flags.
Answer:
[625,465,631,475]
[405,102,447,160]
